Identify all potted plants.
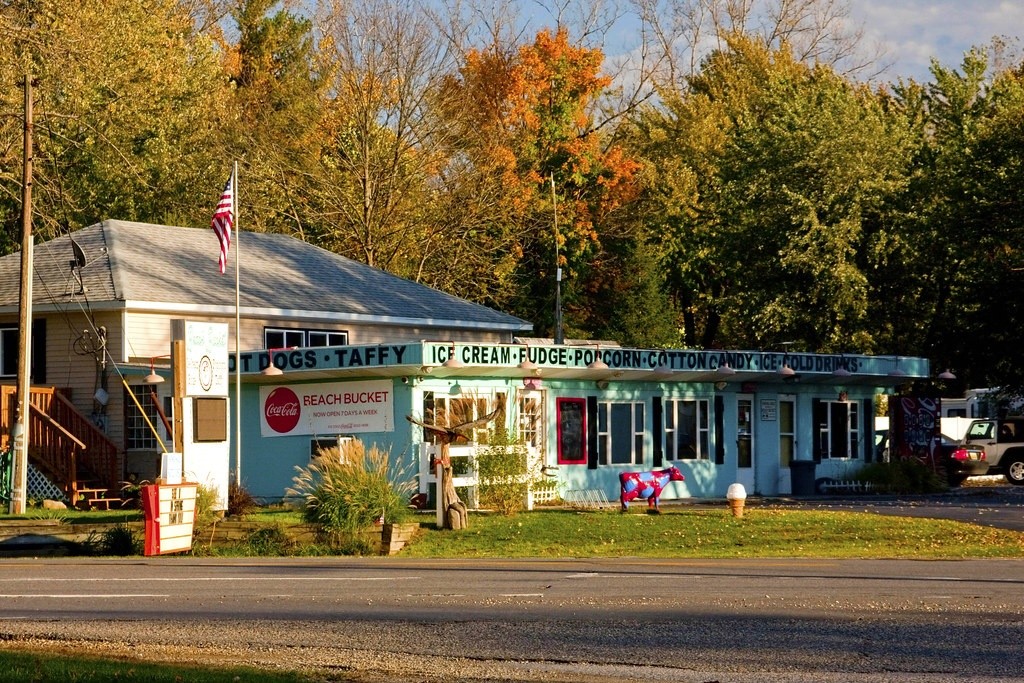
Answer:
[285,435,422,554]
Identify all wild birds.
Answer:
[404,406,506,446]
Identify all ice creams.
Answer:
[726,483,747,518]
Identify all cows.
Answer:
[618,464,686,513]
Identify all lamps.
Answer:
[938,369,956,378]
[143,354,172,383]
[499,342,538,369]
[718,350,736,374]
[574,344,608,369]
[887,355,906,376]
[832,353,851,376]
[655,350,673,375]
[777,351,795,375]
[425,341,464,369]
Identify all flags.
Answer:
[210,166,234,275]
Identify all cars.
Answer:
[875,430,967,489]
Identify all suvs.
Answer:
[949,417,1024,485]
